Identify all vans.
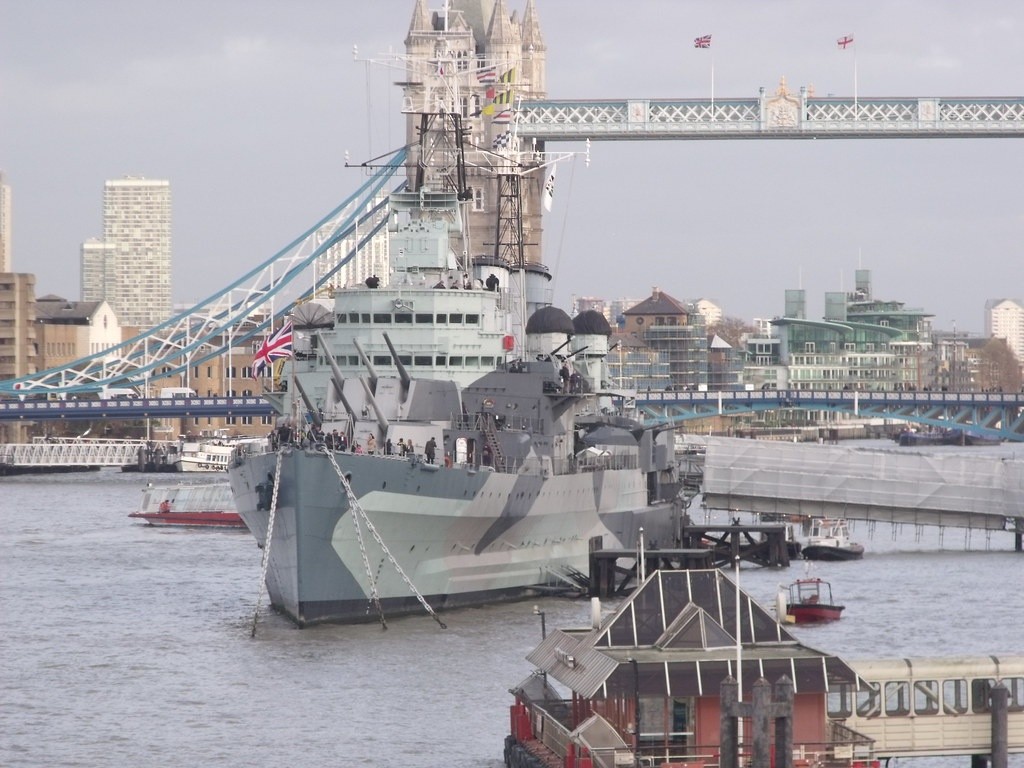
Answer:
[159,388,196,400]
[100,388,141,402]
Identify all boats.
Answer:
[500,565,882,768]
[171,439,235,475]
[129,508,246,530]
[893,425,949,444]
[774,578,847,626]
[944,428,1002,448]
[801,516,866,561]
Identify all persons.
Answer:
[482,444,491,464]
[271,422,413,457]
[560,364,568,393]
[160,500,170,513]
[570,369,579,394]
[425,437,437,464]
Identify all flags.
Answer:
[497,68,514,83]
[492,108,510,124]
[838,33,853,49]
[477,67,496,84]
[492,130,510,147]
[543,163,556,209]
[694,34,711,48]
[492,88,513,104]
[251,320,292,381]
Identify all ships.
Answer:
[217,81,694,628]
